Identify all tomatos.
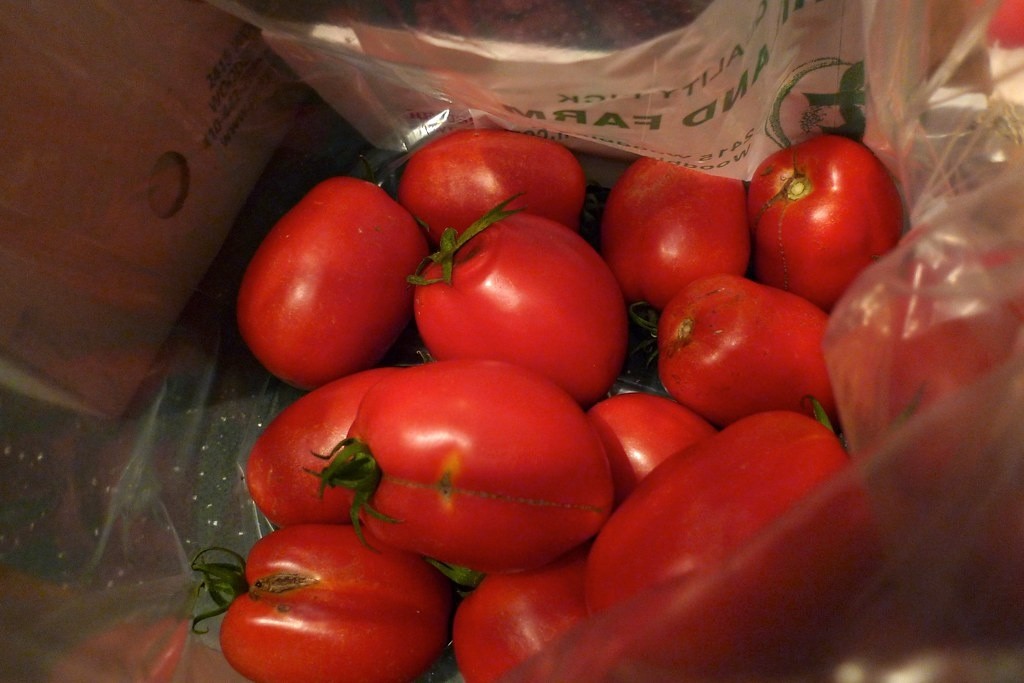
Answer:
[187,135,1024,683]
